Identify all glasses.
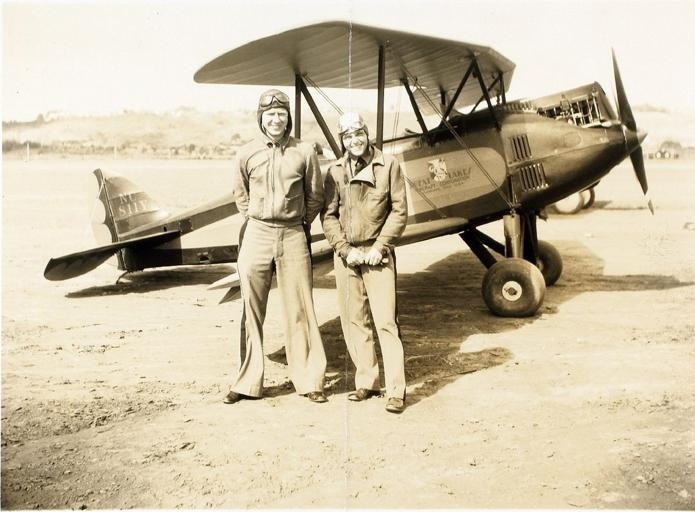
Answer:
[259,93,289,106]
[337,121,364,134]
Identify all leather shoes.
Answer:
[385,395,407,413]
[348,385,381,401]
[303,390,329,403]
[223,390,243,403]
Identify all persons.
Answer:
[317,113,408,413]
[224,90,325,405]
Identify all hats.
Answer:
[336,110,368,142]
[256,89,292,130]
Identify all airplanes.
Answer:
[44,18,658,318]
